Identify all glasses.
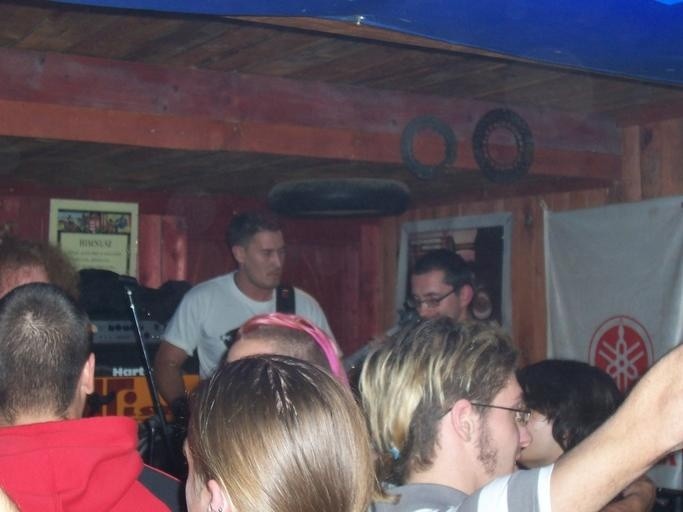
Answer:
[407,288,454,308]
[439,402,530,426]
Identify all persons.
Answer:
[2,241,167,511]
[355,246,682,512]
[183,312,374,511]
[153,211,344,421]
